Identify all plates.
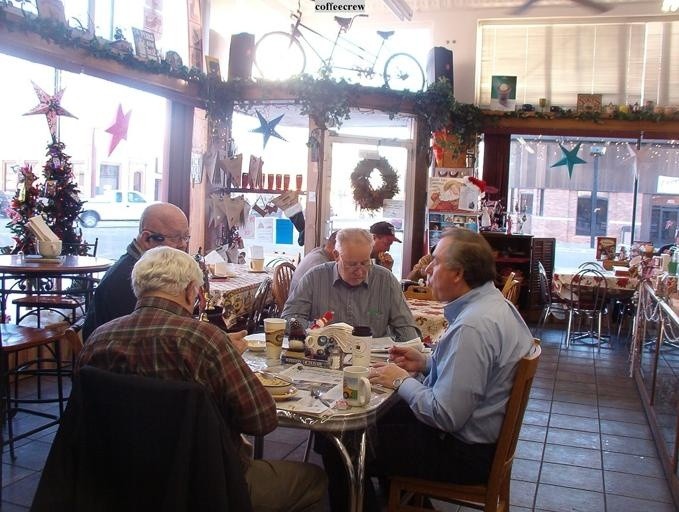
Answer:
[244,339,264,352]
[274,387,299,400]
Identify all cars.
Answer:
[0,190,16,217]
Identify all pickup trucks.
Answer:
[74,190,162,228]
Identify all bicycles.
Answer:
[254,0,427,94]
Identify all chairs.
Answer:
[193,259,522,338]
[534,258,635,353]
[52,365,226,508]
[388,338,541,512]
[0,237,115,463]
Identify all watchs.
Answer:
[392,374,411,391]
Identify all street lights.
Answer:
[589,145,608,248]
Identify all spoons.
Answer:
[312,386,333,410]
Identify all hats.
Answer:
[370,221,401,243]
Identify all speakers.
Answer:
[426,45,455,98]
[227,32,255,81]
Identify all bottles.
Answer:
[328,349,341,370]
[352,326,372,366]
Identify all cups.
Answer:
[251,246,264,270]
[215,262,228,277]
[343,366,372,409]
[263,317,286,361]
[36,241,63,258]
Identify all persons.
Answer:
[362,230,536,507]
[278,227,424,342]
[286,230,337,296]
[368,221,394,276]
[69,246,329,511]
[82,202,192,341]
[406,245,435,288]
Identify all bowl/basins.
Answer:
[501,275,525,285]
[250,371,292,393]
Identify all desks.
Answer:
[193,333,425,512]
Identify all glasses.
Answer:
[145,232,192,241]
[339,255,376,271]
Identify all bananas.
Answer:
[444,180,460,192]
